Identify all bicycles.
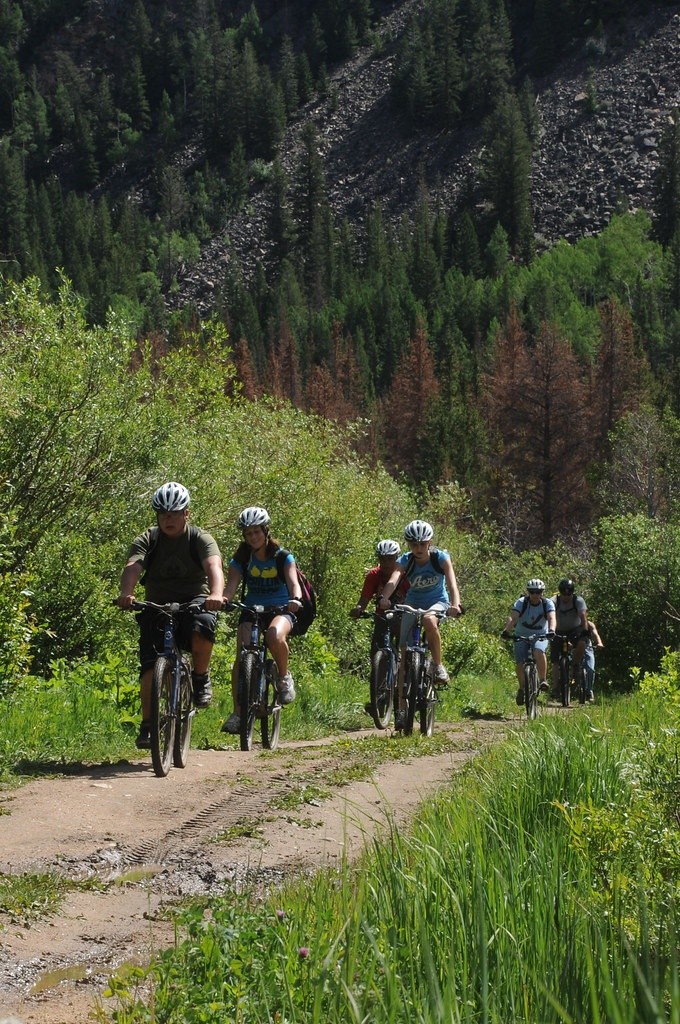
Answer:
[500,632,554,723]
[574,646,600,705]
[382,604,465,741]
[219,597,304,753]
[547,630,590,707]
[111,596,225,776]
[349,610,403,728]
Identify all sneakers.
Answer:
[435,664,449,685]
[277,671,295,704]
[135,720,150,745]
[220,714,239,732]
[191,671,211,706]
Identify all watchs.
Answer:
[291,597,304,604]
[377,594,384,601]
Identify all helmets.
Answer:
[152,482,190,511]
[374,539,400,555]
[559,579,574,594]
[525,578,545,590]
[237,507,268,528]
[404,519,433,542]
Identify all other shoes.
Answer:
[587,691,594,702]
[516,689,524,706]
[540,682,549,691]
[551,679,561,698]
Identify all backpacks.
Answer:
[240,547,317,637]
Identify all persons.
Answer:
[376,520,462,697]
[575,620,604,703]
[118,481,224,749]
[545,577,589,699]
[504,578,557,705]
[350,538,411,718]
[220,504,304,734]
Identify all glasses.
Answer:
[529,589,542,594]
